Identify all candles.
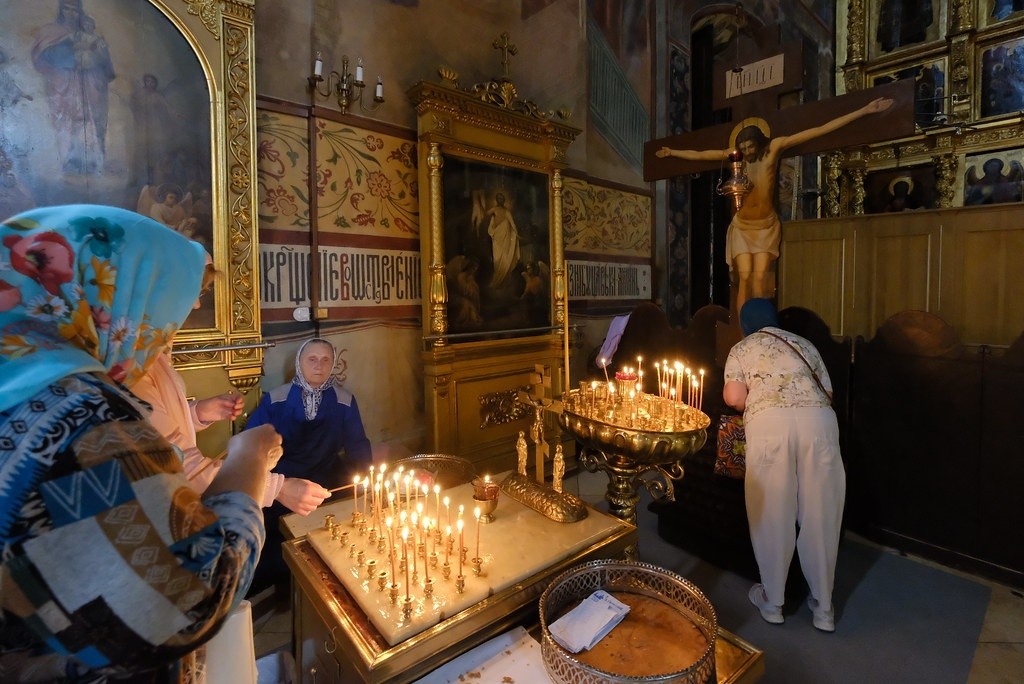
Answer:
[351,462,490,601]
[589,355,708,431]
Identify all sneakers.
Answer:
[807,593,835,631]
[749,584,784,622]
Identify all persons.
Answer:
[0,202,287,683]
[552,444,566,493]
[240,335,374,608]
[122,247,332,526]
[722,297,849,634]
[515,431,529,476]
[526,394,554,445]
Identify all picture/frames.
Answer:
[844,0,1024,215]
[0,0,266,396]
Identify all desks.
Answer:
[280,470,641,684]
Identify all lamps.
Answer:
[716,0,753,210]
[306,49,386,116]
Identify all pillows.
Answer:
[713,413,746,480]
